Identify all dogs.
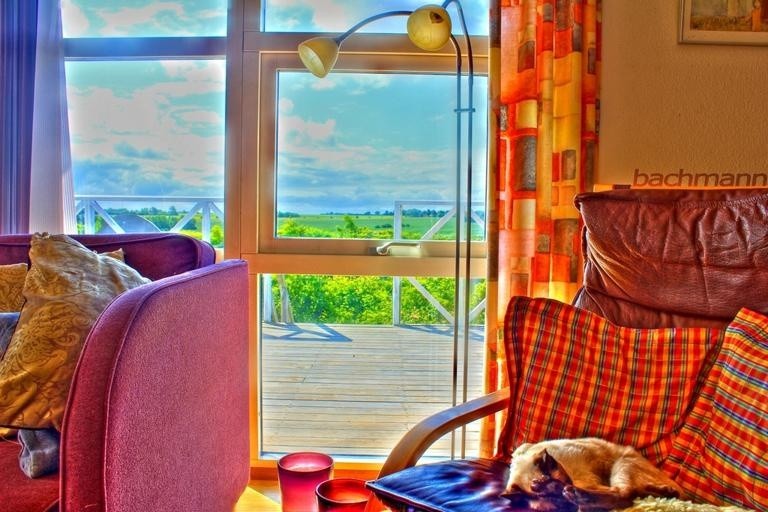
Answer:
[499,437,688,512]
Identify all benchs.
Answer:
[362,180,767,511]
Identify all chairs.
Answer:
[0,233,252,512]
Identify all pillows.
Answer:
[1,238,155,433]
[499,287,767,511]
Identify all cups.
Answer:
[277,449,373,512]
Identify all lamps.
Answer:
[294,0,477,459]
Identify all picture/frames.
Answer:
[677,0,767,49]
